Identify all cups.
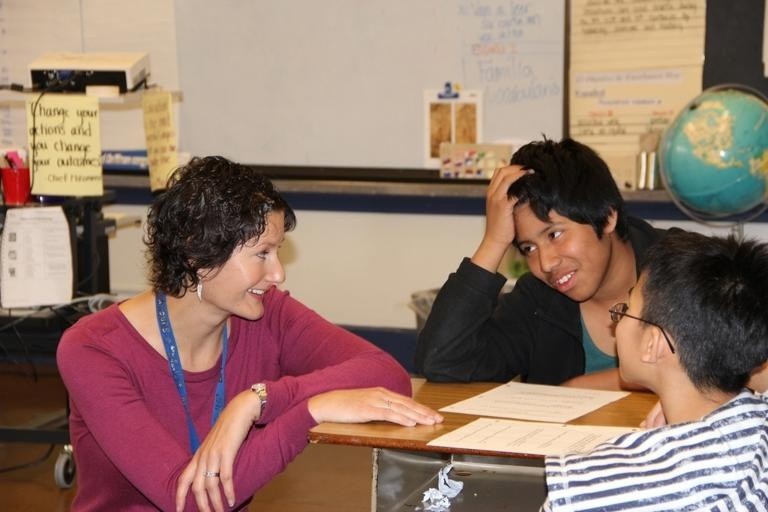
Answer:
[1,168,31,207]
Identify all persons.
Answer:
[56,156,445,511]
[531,229,768,511]
[416,134,683,386]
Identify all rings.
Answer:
[385,399,392,408]
[205,471,221,478]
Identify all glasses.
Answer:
[608,303,676,354]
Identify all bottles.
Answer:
[635,150,657,192]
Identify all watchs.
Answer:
[251,381,269,414]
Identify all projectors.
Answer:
[30,52,150,95]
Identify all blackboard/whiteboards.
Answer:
[0,0,570,187]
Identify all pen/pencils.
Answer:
[2,148,30,171]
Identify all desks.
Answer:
[308,377,664,512]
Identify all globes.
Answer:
[657,83,768,244]
